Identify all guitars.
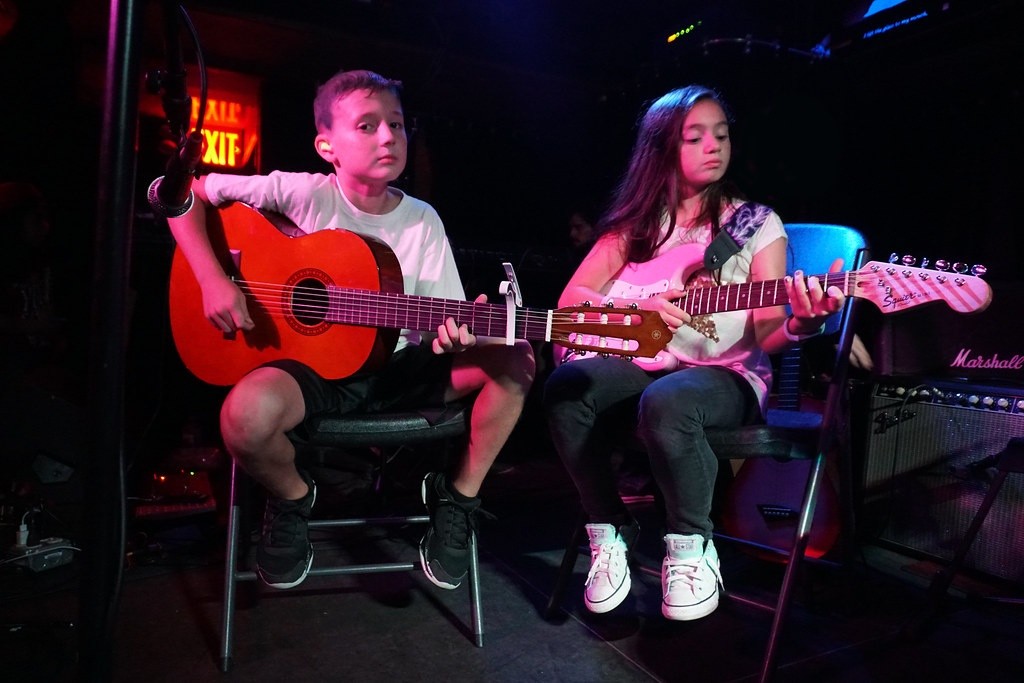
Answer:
[551,239,995,378]
[166,197,676,392]
[719,341,856,581]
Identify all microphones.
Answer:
[148,130,206,218]
[977,436,1024,470]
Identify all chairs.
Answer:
[218,411,486,673]
[545,225,867,682]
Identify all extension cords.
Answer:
[6,536,74,573]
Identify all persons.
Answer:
[163,71,537,590]
[542,86,845,621]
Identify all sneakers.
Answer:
[254,474,316,590]
[422,472,496,590]
[661,531,724,619]
[584,517,640,613]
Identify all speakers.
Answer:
[855,372,1024,590]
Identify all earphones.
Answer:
[319,142,333,152]
